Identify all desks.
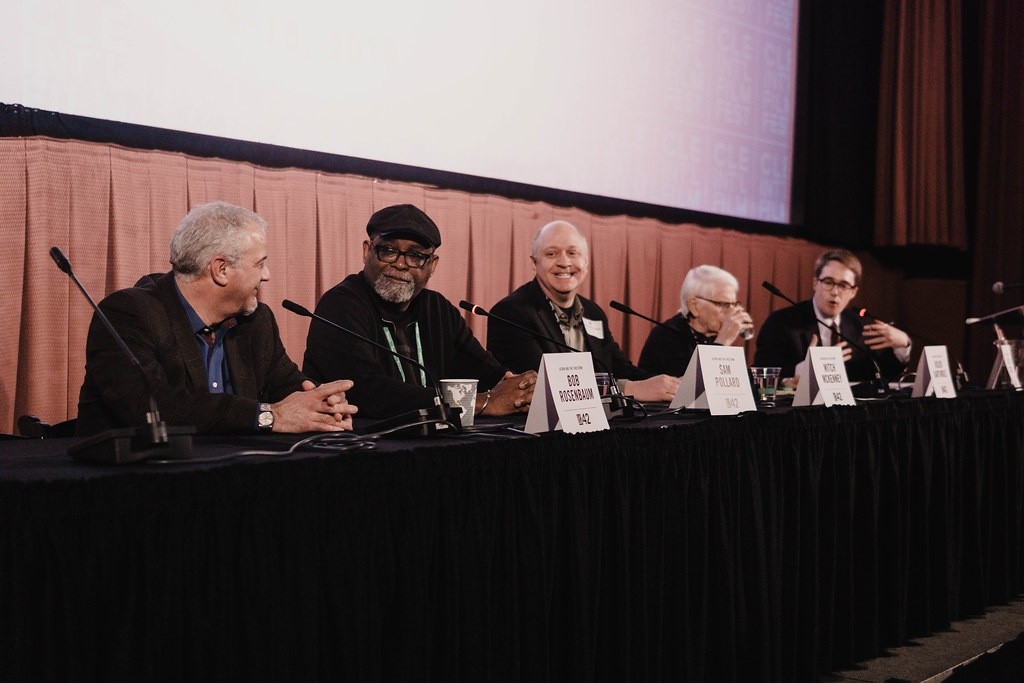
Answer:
[0,379,1024,682]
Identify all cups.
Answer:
[738,322,754,340]
[595,372,614,399]
[434,379,479,426]
[750,366,782,407]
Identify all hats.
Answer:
[366,203,441,247]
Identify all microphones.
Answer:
[610,300,705,344]
[49,247,196,466]
[759,281,891,399]
[966,306,1024,325]
[281,299,463,439]
[459,301,636,421]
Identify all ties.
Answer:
[830,323,840,346]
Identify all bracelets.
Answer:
[473,388,491,419]
[615,379,628,397]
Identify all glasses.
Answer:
[372,243,434,269]
[695,293,738,309]
[818,275,857,294]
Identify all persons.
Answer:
[486,219,682,402]
[300,201,537,425]
[751,249,912,388]
[632,264,758,395]
[76,201,358,433]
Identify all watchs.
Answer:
[258,401,275,431]
